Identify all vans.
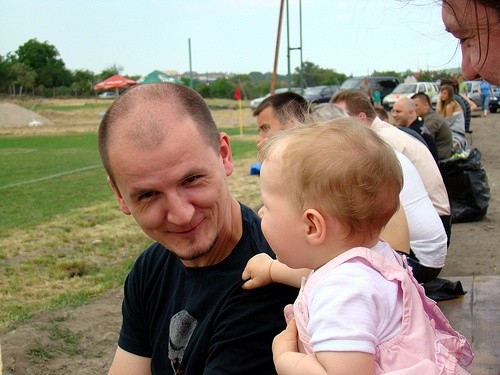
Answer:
[339,75,400,106]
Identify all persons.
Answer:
[436,74,478,153]
[253,91,411,272]
[241,97,476,375]
[392,95,439,168]
[329,89,451,250]
[374,108,430,152]
[411,92,453,161]
[358,77,374,106]
[478,74,493,117]
[308,103,447,283]
[97,82,302,375]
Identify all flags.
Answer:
[235,78,242,101]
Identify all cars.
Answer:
[301,85,341,104]
[461,80,500,114]
[250,87,304,111]
[382,82,440,112]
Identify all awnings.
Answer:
[93,73,138,99]
[136,70,185,87]
